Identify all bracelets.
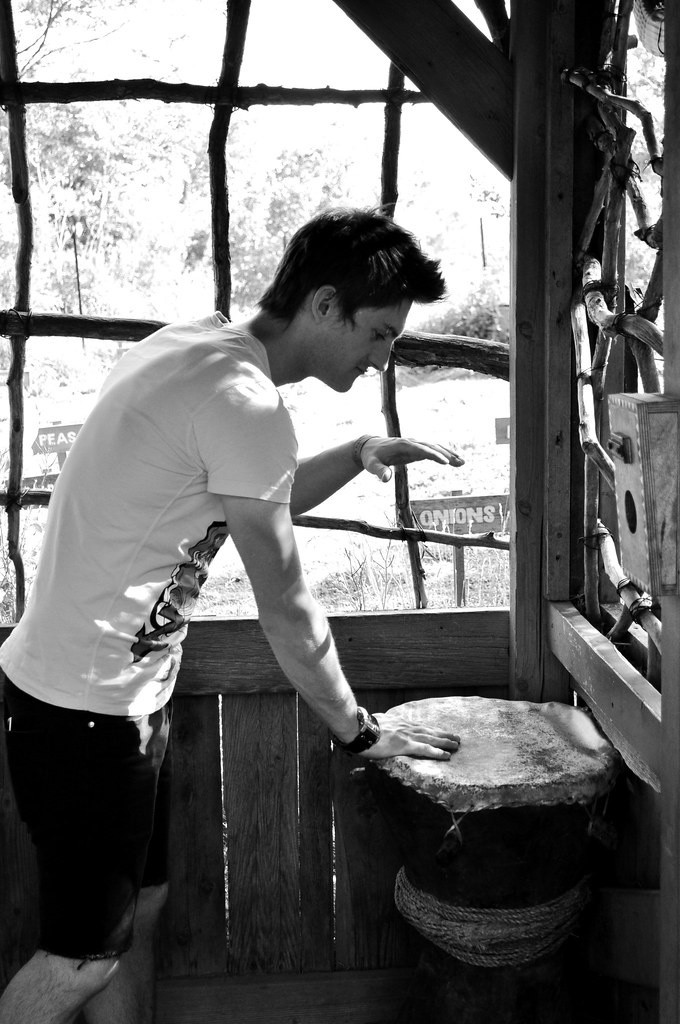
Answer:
[350,434,383,471]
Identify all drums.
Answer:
[351,690,613,1024]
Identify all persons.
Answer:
[0,210,463,1024]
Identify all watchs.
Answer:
[326,705,379,756]
